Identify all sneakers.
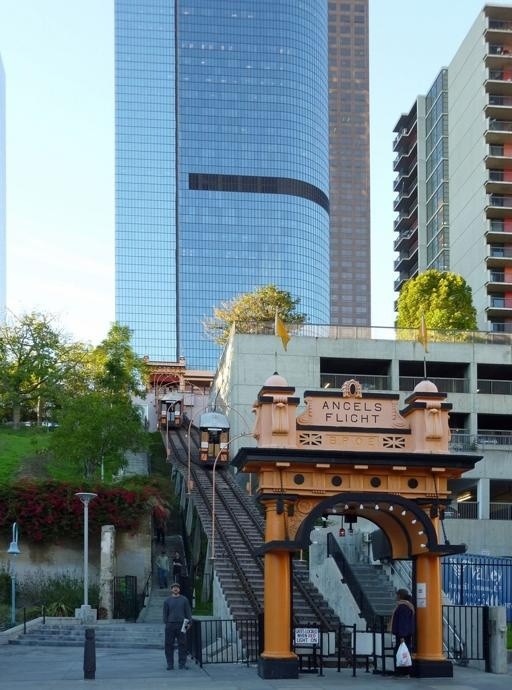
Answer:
[178,664,189,670]
[159,584,168,589]
[392,674,411,679]
[166,666,173,670]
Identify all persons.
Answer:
[155,550,170,589]
[155,507,169,547]
[161,583,193,672]
[171,551,184,583]
[386,589,415,677]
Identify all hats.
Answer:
[396,588,412,598]
[170,583,182,589]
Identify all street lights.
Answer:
[7,522,22,625]
[75,492,98,679]
